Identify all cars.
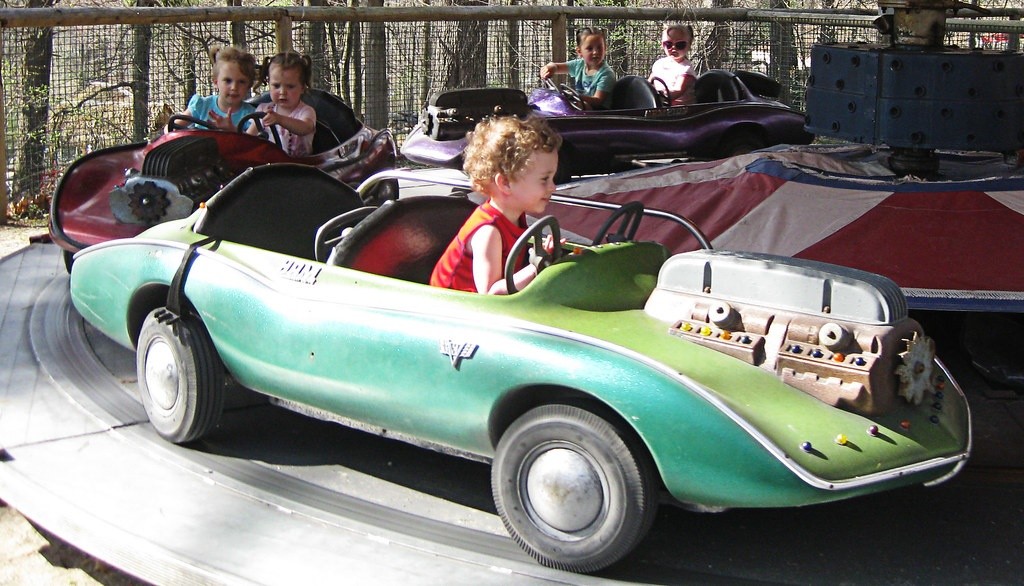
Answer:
[46,88,399,275]
[67,164,971,576]
[399,68,818,170]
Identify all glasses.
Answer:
[662,38,692,51]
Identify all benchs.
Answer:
[696,69,740,101]
[246,89,361,152]
[194,163,366,263]
[324,195,479,282]
[611,75,658,114]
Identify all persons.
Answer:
[163,47,262,135]
[207,52,316,156]
[647,23,696,106]
[540,27,615,111]
[429,116,566,295]
